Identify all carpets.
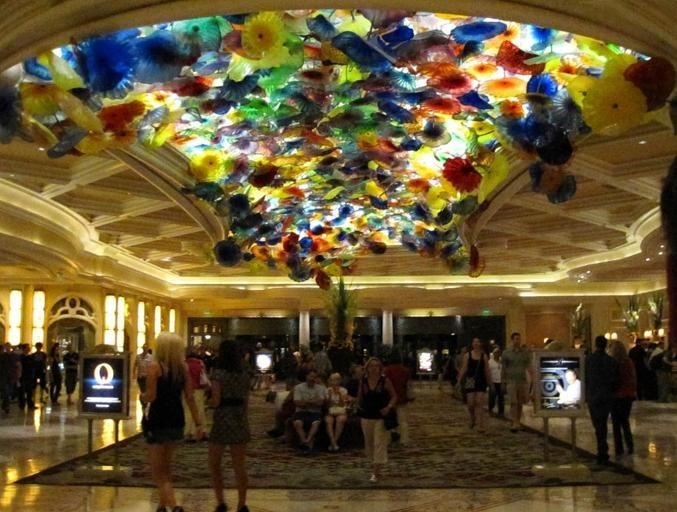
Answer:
[12,380,666,491]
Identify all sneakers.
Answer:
[328,445,339,450]
[368,475,377,483]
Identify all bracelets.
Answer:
[193,422,203,428]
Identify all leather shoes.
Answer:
[216,503,249,512]
[156,506,184,512]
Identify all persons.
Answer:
[1,336,264,414]
[356,351,398,486]
[204,339,253,512]
[139,332,203,512]
[182,345,208,443]
[265,331,676,465]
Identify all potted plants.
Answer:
[321,271,360,386]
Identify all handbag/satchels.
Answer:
[385,412,398,430]
[142,406,156,444]
[329,406,345,416]
